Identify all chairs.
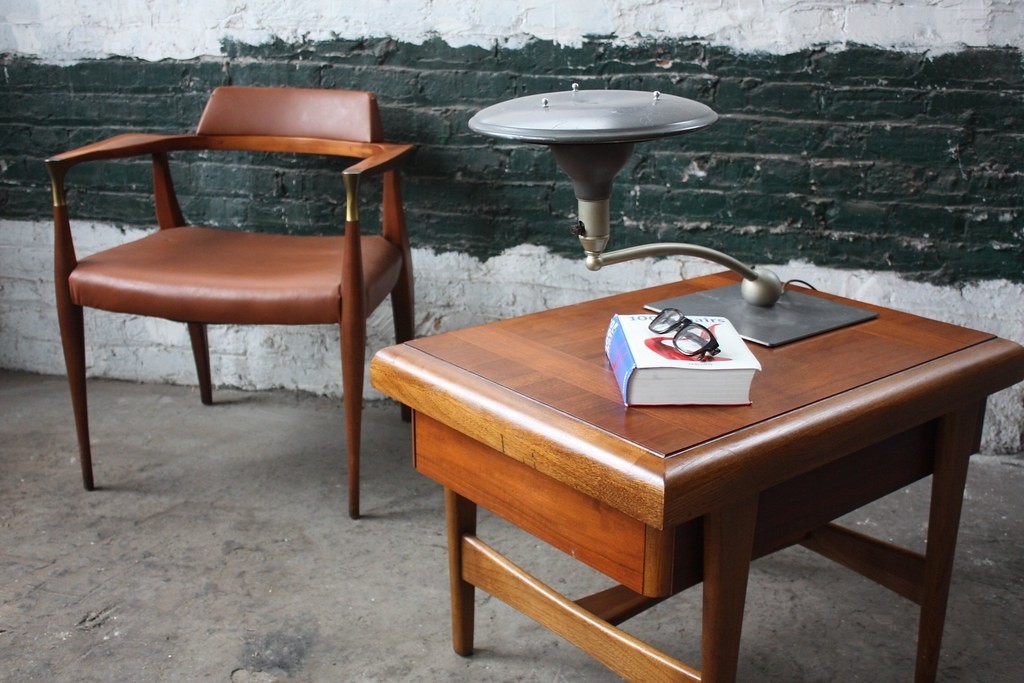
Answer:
[42,88,416,519]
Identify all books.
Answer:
[605,312,762,406]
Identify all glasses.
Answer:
[649,308,721,360]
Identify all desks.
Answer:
[369,269,1023,683]
